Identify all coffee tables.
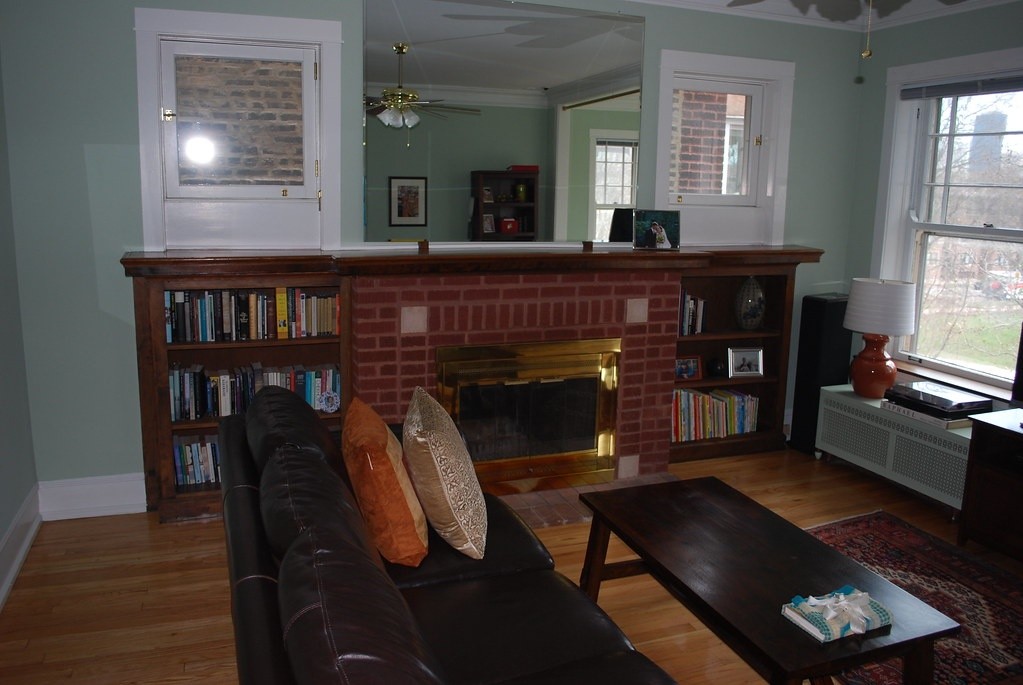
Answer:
[578,475,961,685]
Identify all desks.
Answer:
[815,384,972,511]
[953,407,1023,560]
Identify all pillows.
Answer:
[342,397,429,566]
[403,385,487,560]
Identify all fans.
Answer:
[365,42,481,119]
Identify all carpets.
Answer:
[805,509,1023,685]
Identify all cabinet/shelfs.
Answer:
[133,273,352,523]
[671,263,796,462]
[470,170,539,241]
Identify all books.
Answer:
[708,388,759,439]
[781,584,894,644]
[164,290,276,343]
[293,362,341,410]
[234,361,295,416]
[881,381,994,430]
[677,279,707,338]
[173,434,221,486]
[672,388,719,444]
[276,288,340,339]
[169,359,235,422]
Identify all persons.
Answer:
[739,357,756,372]
[645,221,671,249]
[677,360,694,379]
[484,216,492,231]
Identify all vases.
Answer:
[735,276,764,330]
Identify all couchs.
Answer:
[216,385,676,685]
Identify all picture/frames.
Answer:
[483,214,495,233]
[728,346,765,378]
[632,209,680,250]
[483,187,494,203]
[388,176,428,226]
[675,354,702,382]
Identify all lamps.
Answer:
[377,107,420,128]
[842,277,914,399]
